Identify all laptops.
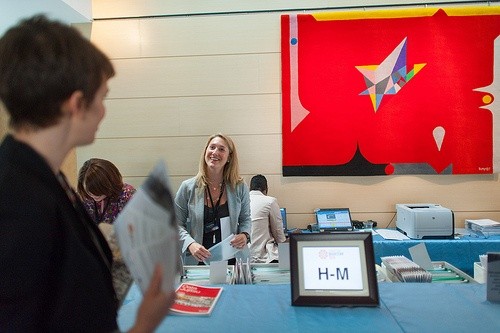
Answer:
[280,208,287,233]
[314,207,354,232]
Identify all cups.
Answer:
[363,222,373,233]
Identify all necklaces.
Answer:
[206,182,223,207]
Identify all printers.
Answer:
[395,203,455,240]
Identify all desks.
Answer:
[117,277,500,333]
[373,228,500,275]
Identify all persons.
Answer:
[0,14,119,333]
[236,175,285,265]
[175,134,250,266]
[75,158,140,223]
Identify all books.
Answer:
[170,284,226,315]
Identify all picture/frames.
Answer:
[289,232,380,307]
